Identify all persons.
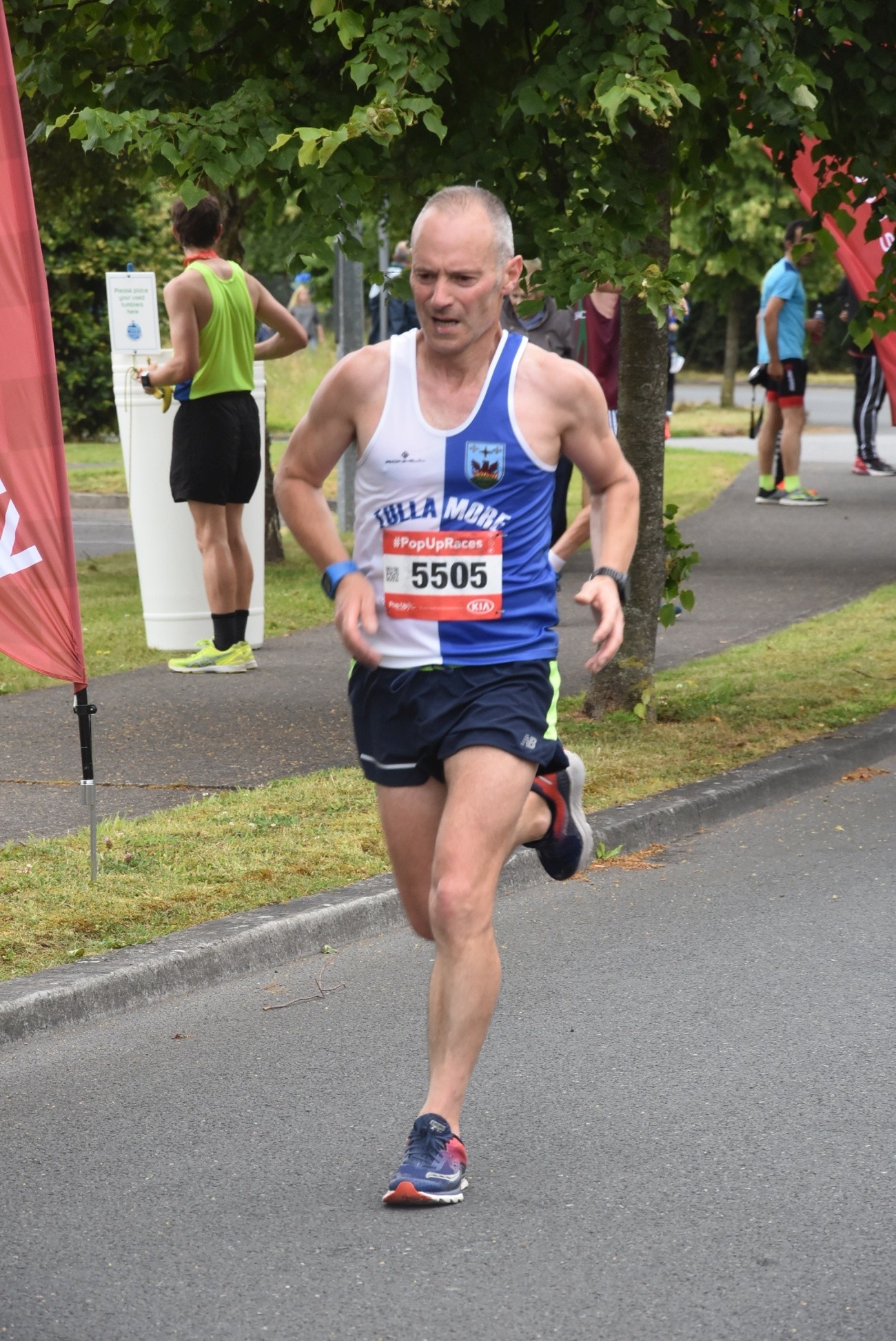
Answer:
[500,258,689,590]
[137,186,309,674]
[274,184,643,1207]
[756,220,896,506]
[287,238,423,346]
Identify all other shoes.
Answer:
[658,604,683,621]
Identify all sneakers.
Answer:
[521,751,594,880]
[383,1111,468,1205]
[754,486,782,504]
[236,641,257,669]
[779,487,829,506]
[852,454,896,477]
[167,636,248,674]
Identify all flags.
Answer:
[0,0,86,686]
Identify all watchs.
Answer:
[321,561,357,600]
[141,371,153,389]
[589,567,633,605]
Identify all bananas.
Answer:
[134,357,174,413]
